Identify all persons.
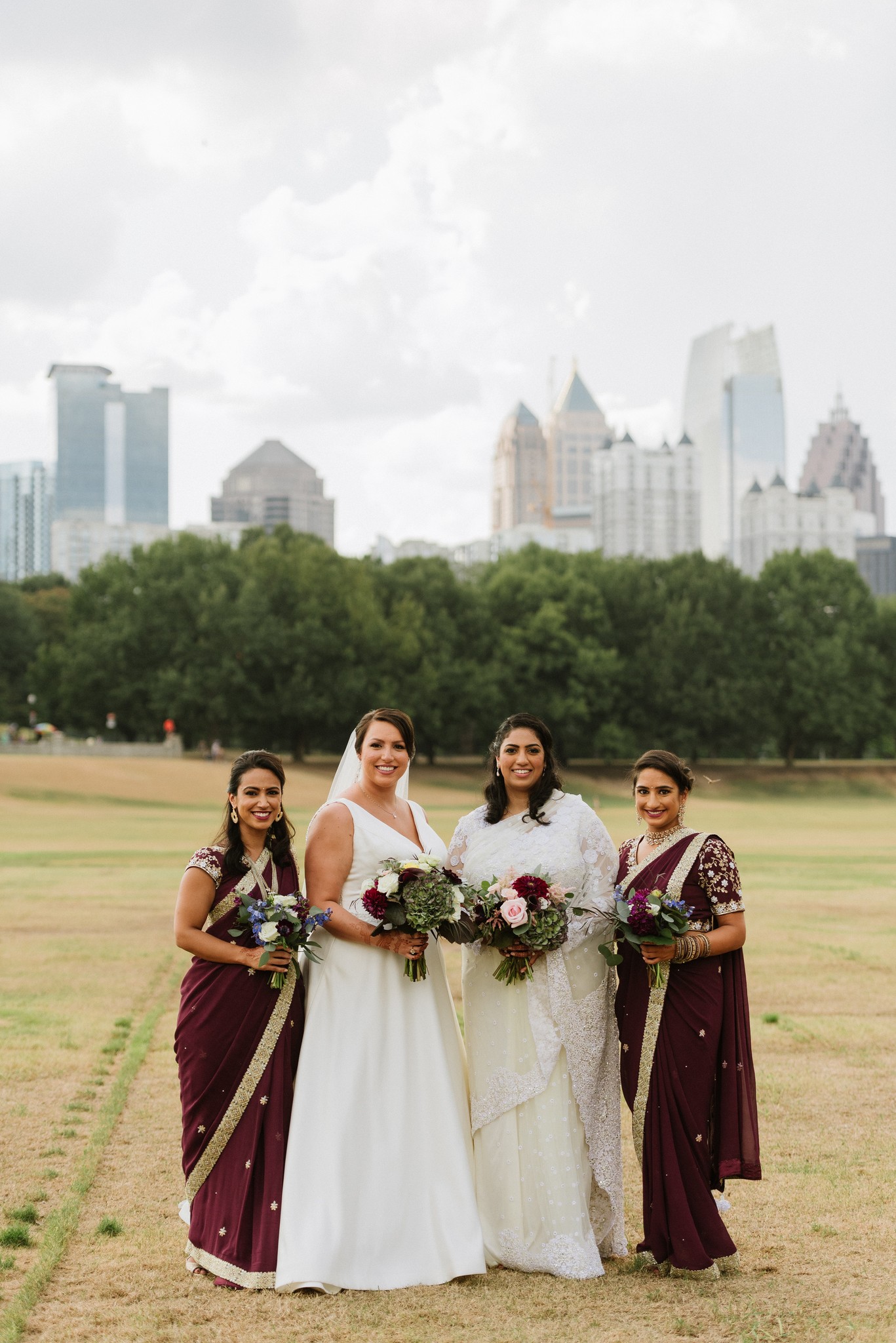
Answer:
[597,751,768,1282]
[274,705,491,1298]
[433,705,630,1281]
[11,692,232,759]
[172,746,311,1291]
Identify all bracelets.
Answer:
[669,932,713,965]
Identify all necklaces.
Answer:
[505,806,516,817]
[359,778,398,819]
[644,823,684,845]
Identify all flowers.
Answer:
[566,883,698,988]
[347,845,474,983]
[232,884,331,989]
[470,861,576,986]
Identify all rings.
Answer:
[532,951,535,957]
[409,946,416,955]
